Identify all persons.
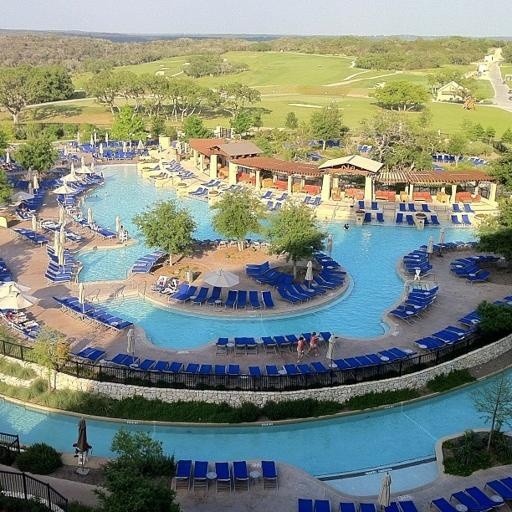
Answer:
[154,278,164,290]
[296,337,304,363]
[306,335,318,356]
[163,281,175,295]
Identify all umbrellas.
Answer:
[305,260,313,288]
[76,418,90,452]
[378,472,391,506]
[204,270,240,287]
[326,334,339,359]
[426,236,433,268]
[0,130,144,314]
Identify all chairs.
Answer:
[389,239,512,353]
[142,159,194,180]
[1,158,134,366]
[70,139,177,160]
[173,459,278,495]
[283,138,490,170]
[298,476,512,512]
[188,179,321,213]
[132,238,348,312]
[359,201,473,225]
